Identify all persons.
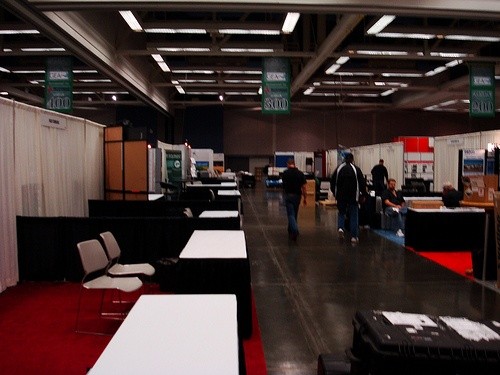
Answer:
[381,178,407,237]
[263,162,272,182]
[330,153,366,243]
[442,183,461,208]
[281,160,307,240]
[371,159,388,195]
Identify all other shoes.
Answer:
[350,237,359,242]
[339,228,345,239]
[391,207,401,214]
[396,229,404,237]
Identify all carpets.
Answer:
[404,246,474,282]
[0,283,268,375]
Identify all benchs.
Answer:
[376,196,445,231]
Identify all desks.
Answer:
[178,230,253,341]
[196,209,240,230]
[406,207,486,252]
[217,189,244,216]
[84,293,247,375]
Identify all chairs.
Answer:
[73,239,143,337]
[208,189,215,209]
[100,231,155,315]
[180,206,195,239]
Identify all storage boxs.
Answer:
[346,308,500,375]
